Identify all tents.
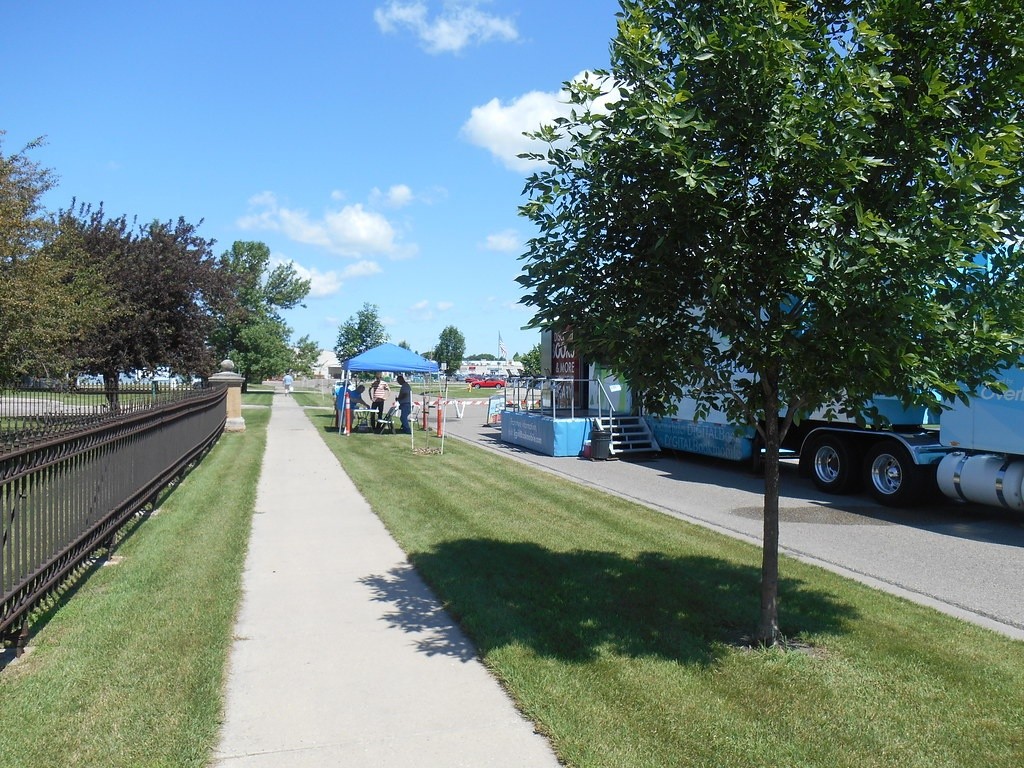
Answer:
[329,343,446,436]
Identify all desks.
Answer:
[354,410,379,433]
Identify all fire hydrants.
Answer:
[467,385,474,393]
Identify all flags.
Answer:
[500,335,507,357]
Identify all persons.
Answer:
[369,372,391,433]
[333,380,370,433]
[282,373,293,398]
[395,376,412,434]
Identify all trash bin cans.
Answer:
[591,430,612,459]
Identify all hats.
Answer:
[342,380,351,385]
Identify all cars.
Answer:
[472,378,505,389]
[332,379,355,397]
[466,377,478,383]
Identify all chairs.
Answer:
[356,405,369,426]
[376,407,398,434]
[400,405,420,431]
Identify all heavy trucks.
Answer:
[589,293,1024,521]
